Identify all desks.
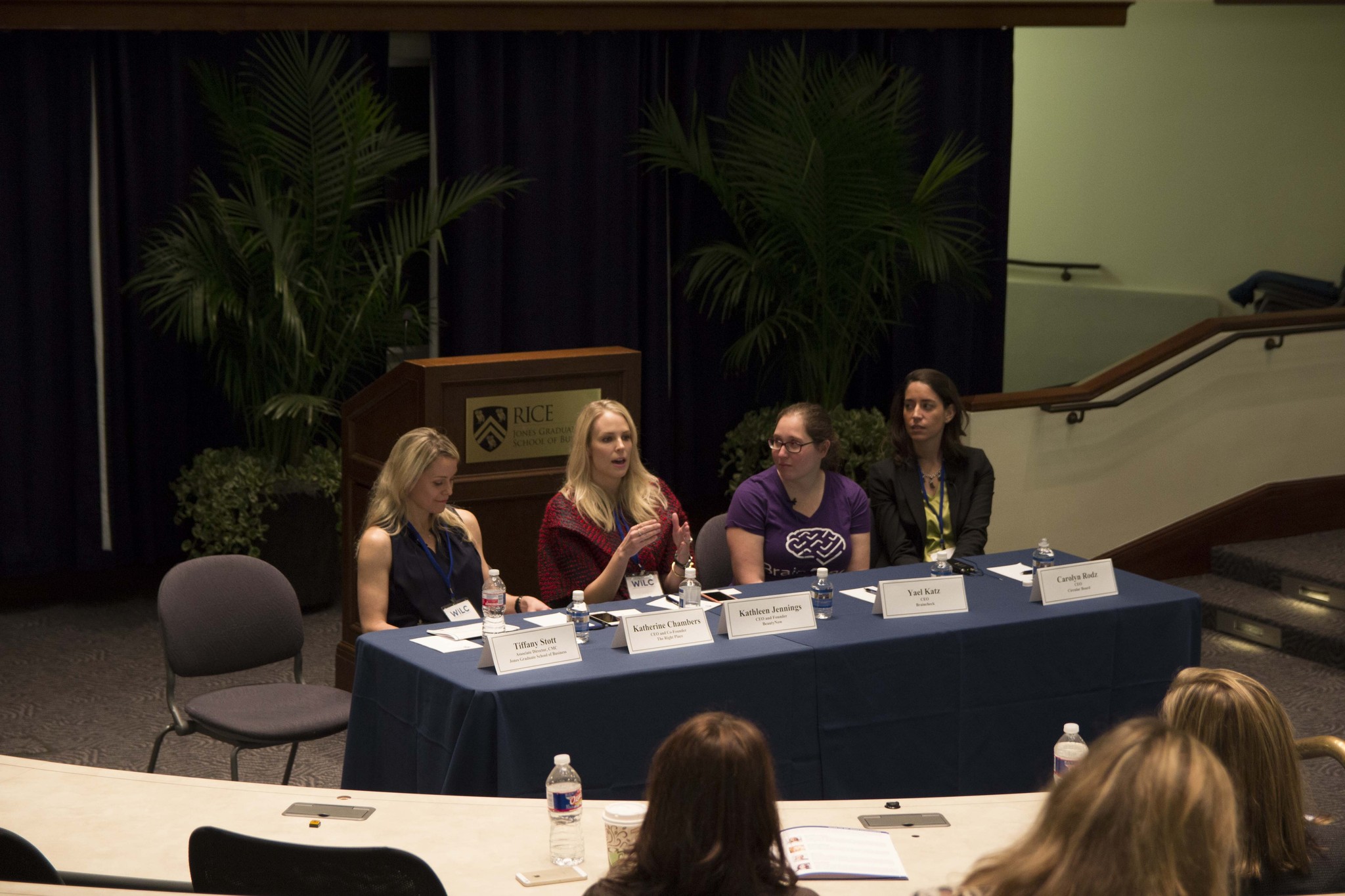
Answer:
[342,547,1202,799]
[0,755,1050,896]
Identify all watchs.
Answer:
[674,550,693,570]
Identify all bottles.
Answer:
[810,567,833,619]
[1032,538,1056,580]
[1054,723,1088,783]
[679,567,702,608]
[931,551,953,577]
[482,569,506,643]
[545,754,585,866]
[566,590,590,644]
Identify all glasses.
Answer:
[767,438,822,453]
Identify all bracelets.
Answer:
[671,562,686,578]
[514,595,523,613]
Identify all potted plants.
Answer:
[122,30,533,605]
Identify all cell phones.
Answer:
[516,866,588,887]
[589,611,620,626]
[701,589,739,605]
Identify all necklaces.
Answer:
[920,467,940,490]
[419,531,437,546]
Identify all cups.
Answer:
[601,801,647,869]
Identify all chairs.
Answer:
[695,513,733,591]
[148,554,353,786]
[188,825,447,896]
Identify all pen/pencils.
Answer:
[665,595,679,605]
[1020,570,1032,575]
[863,587,877,594]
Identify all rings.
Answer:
[683,536,693,544]
[638,529,641,535]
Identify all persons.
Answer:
[912,667,1345,896]
[725,401,872,586]
[540,401,698,608]
[583,712,820,896]
[866,368,995,567]
[353,428,555,633]
